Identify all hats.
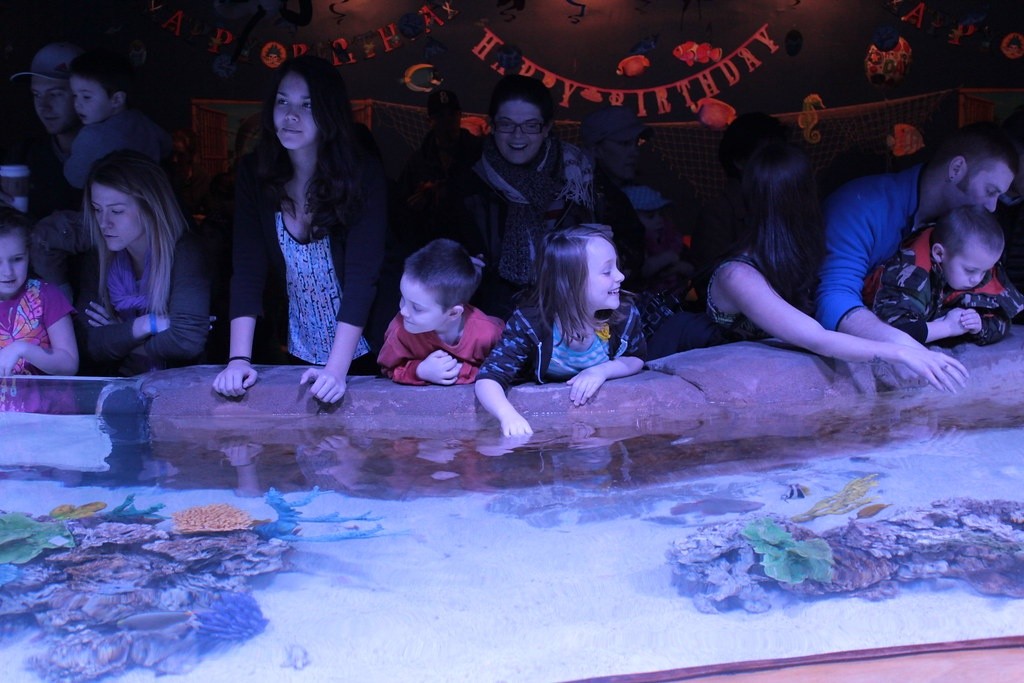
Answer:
[427,89,461,117]
[581,107,654,145]
[9,41,88,82]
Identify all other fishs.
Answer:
[116,610,195,631]
[671,483,812,516]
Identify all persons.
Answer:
[0,38,1023,440]
